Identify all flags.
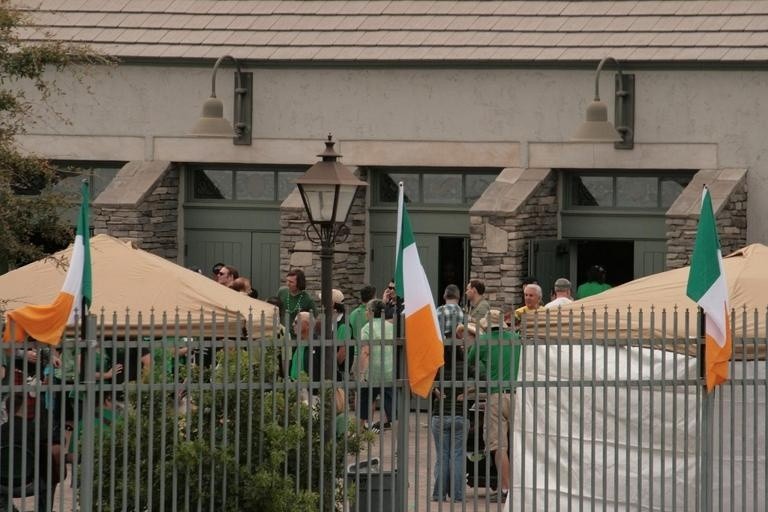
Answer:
[687,186,732,393]
[5,183,91,352]
[394,204,446,399]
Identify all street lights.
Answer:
[285,127,374,512]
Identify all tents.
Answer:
[503,241,768,512]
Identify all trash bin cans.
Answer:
[347,457,400,512]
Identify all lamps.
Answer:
[567,54,635,151]
[183,54,253,149]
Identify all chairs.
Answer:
[0,443,50,512]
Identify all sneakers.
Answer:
[363,424,385,435]
[379,420,397,430]
[488,489,511,503]
[450,497,470,503]
[433,498,448,502]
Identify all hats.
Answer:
[319,289,345,304]
[479,309,508,329]
[455,322,484,338]
